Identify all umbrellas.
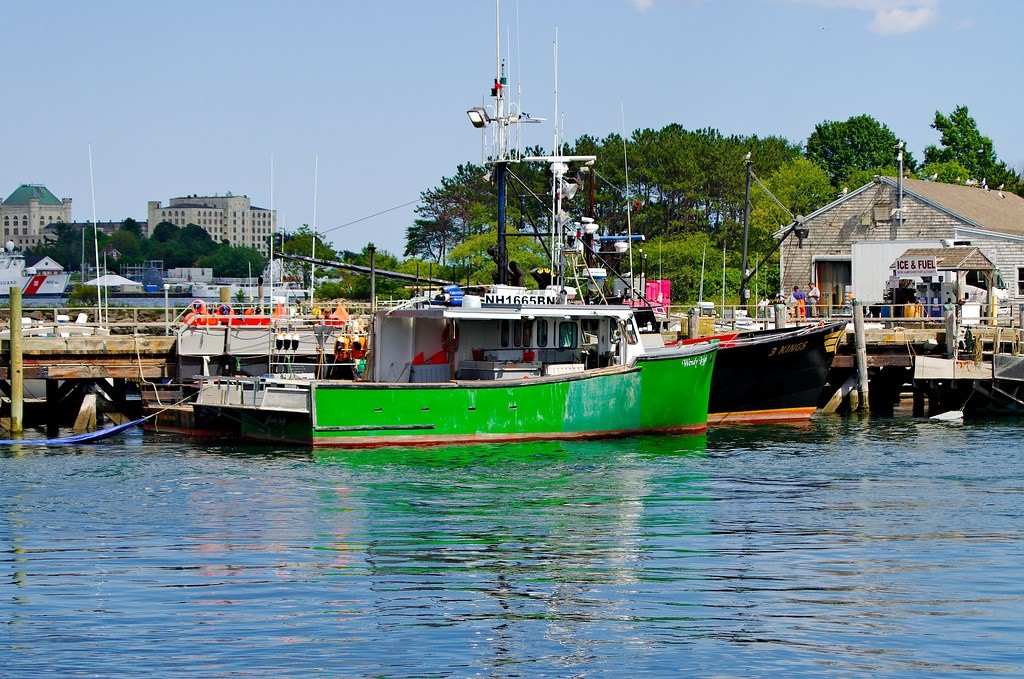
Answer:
[83,273,143,287]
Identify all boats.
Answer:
[1,144,128,401]
[0,241,72,298]
[140,2,852,448]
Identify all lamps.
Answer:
[467,107,491,129]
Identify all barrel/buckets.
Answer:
[145,285,158,292]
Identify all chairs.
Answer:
[22,313,94,338]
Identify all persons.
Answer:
[362,326,369,351]
[792,281,820,318]
